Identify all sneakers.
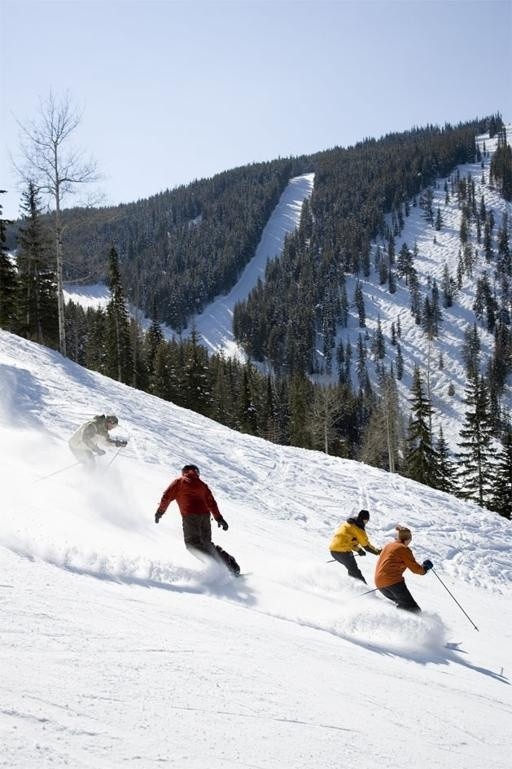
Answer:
[229,557,240,577]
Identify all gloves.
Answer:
[422,560,433,575]
[94,447,105,456]
[216,518,228,531]
[116,440,127,446]
[155,510,163,523]
[358,549,366,556]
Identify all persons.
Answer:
[372,526,434,614]
[329,509,383,584]
[68,413,127,468]
[155,464,229,566]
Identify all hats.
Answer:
[359,510,369,521]
[399,527,411,541]
